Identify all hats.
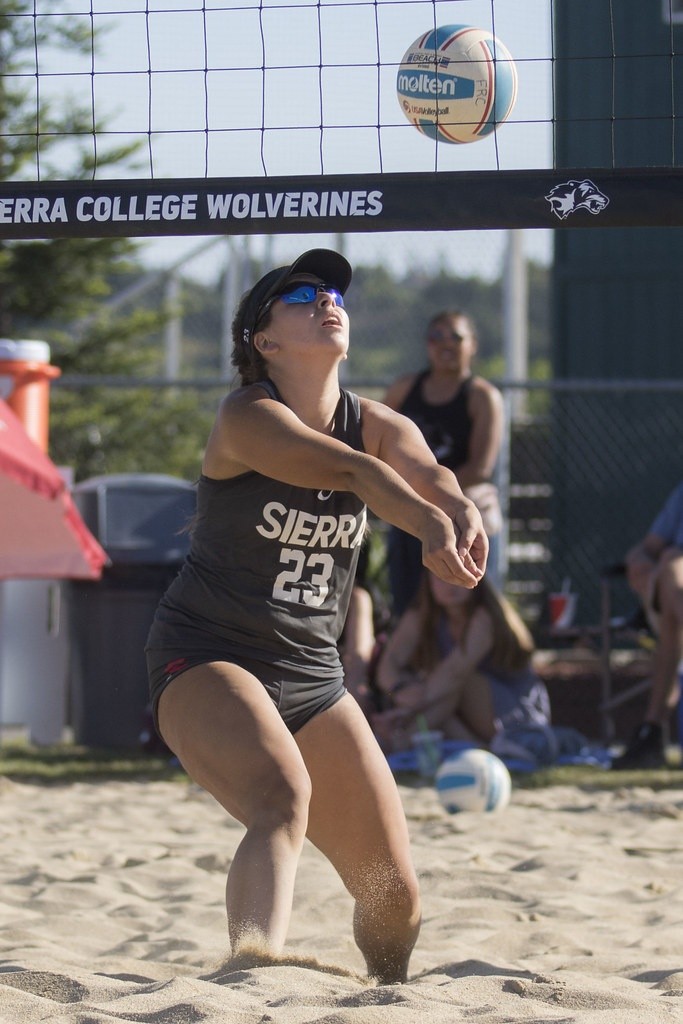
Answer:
[239,249,351,374]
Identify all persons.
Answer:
[339,583,372,712]
[622,478,683,750]
[374,552,557,762]
[146,266,491,983]
[383,309,507,610]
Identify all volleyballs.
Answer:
[395,20,520,144]
[434,750,513,814]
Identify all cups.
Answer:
[548,592,578,634]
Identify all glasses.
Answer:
[427,330,463,346]
[255,282,348,325]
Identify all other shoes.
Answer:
[612,724,665,771]
